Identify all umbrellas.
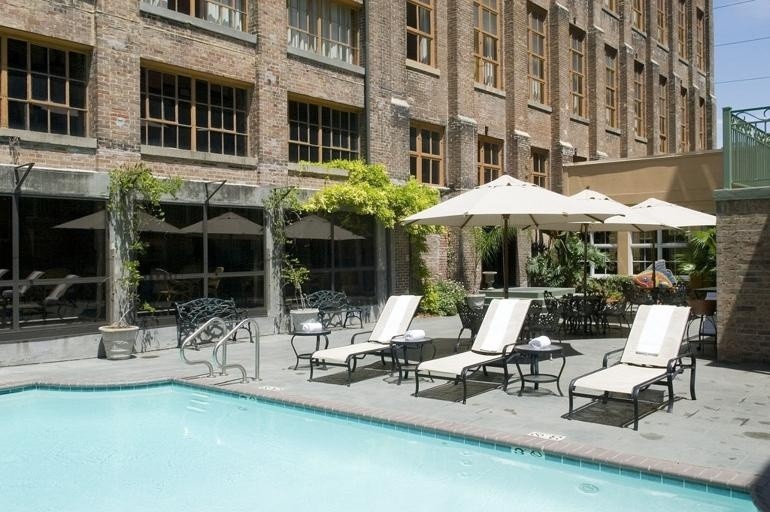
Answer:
[629,197,716,304]
[283,214,367,241]
[53,208,180,234]
[521,188,682,342]
[179,212,264,297]
[400,174,626,298]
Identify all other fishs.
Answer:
[633,260,677,289]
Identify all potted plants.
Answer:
[97,159,184,361]
[278,254,319,332]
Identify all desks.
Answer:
[390,335,437,385]
[515,344,566,396]
[291,330,331,371]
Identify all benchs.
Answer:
[302,289,364,330]
[171,297,253,350]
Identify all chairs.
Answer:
[414,298,534,405]
[18,260,226,326]
[455,280,716,362]
[569,305,698,431]
[308,294,426,387]
